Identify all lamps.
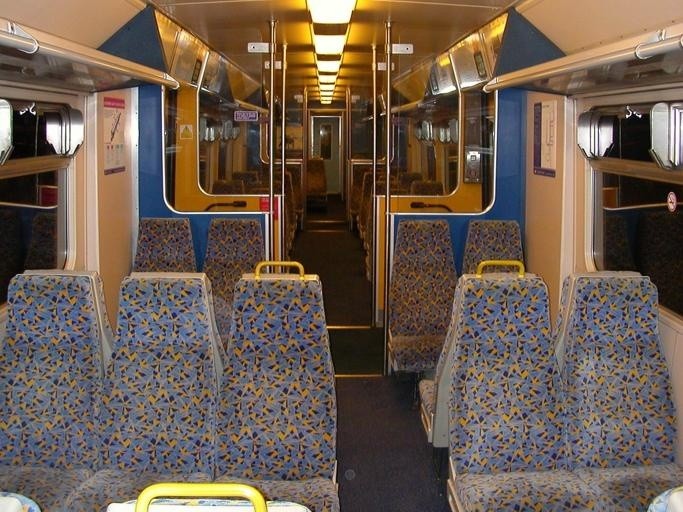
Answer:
[306,0,357,105]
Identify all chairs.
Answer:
[447,277,597,512]
[307,159,327,211]
[350,164,443,282]
[0,208,24,291]
[0,270,113,512]
[419,260,538,448]
[604,214,661,305]
[637,211,683,314]
[202,219,265,352]
[213,167,310,261]
[66,272,224,512]
[553,271,643,371]
[3,212,58,301]
[552,277,683,512]
[133,218,196,275]
[462,220,526,275]
[214,262,340,512]
[389,219,460,373]
